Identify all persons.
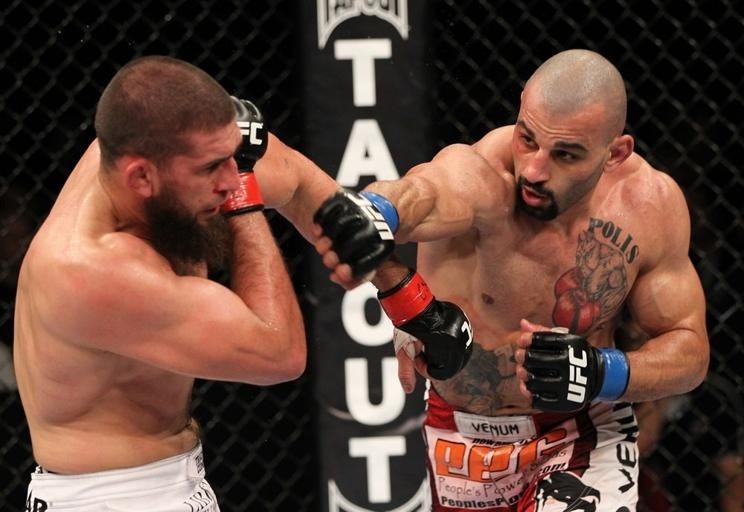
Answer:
[635,385,744,512]
[311,47,712,511]
[12,56,473,512]
